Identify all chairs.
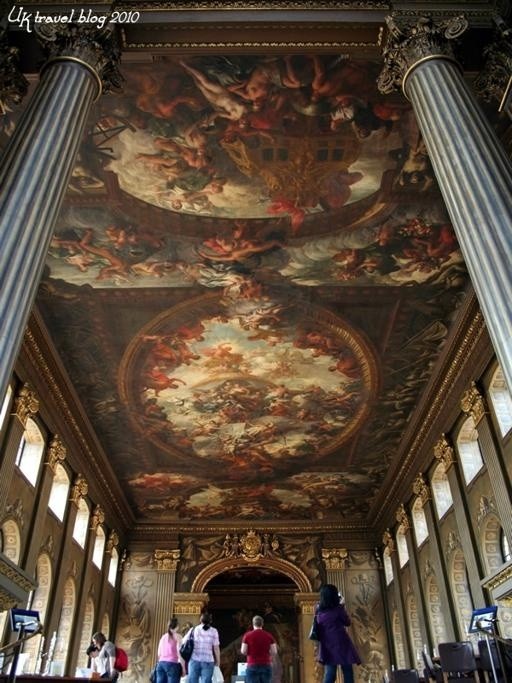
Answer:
[385,639,512,683]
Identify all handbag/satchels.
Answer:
[150,662,160,681]
[308,608,322,641]
[178,626,196,661]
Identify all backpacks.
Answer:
[113,646,131,672]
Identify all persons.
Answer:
[86,644,109,678]
[240,614,278,683]
[53,56,470,517]
[154,618,187,683]
[177,609,221,683]
[91,631,120,682]
[312,582,362,683]
